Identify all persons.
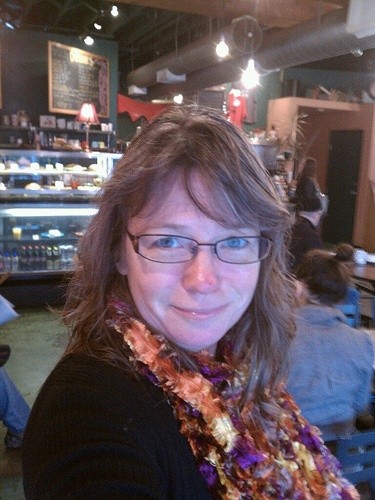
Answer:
[250,243,375,485]
[0,345,33,449]
[285,157,330,268]
[22,105,360,500]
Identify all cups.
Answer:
[12,227,22,241]
[354,250,367,265]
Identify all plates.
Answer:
[41,232,65,237]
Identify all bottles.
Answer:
[5,244,58,272]
[268,125,278,138]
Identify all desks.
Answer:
[307,249,375,298]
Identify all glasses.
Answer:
[122,223,275,265]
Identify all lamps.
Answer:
[75,101,100,154]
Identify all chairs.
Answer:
[334,432,375,500]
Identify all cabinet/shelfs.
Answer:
[0,205,98,275]
[0,125,123,208]
